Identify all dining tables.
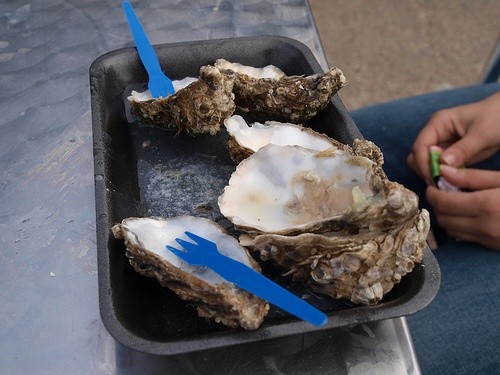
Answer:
[0,0,425,375]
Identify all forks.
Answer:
[120,0,176,97]
[166,231,328,327]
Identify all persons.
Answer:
[349,81,500,375]
